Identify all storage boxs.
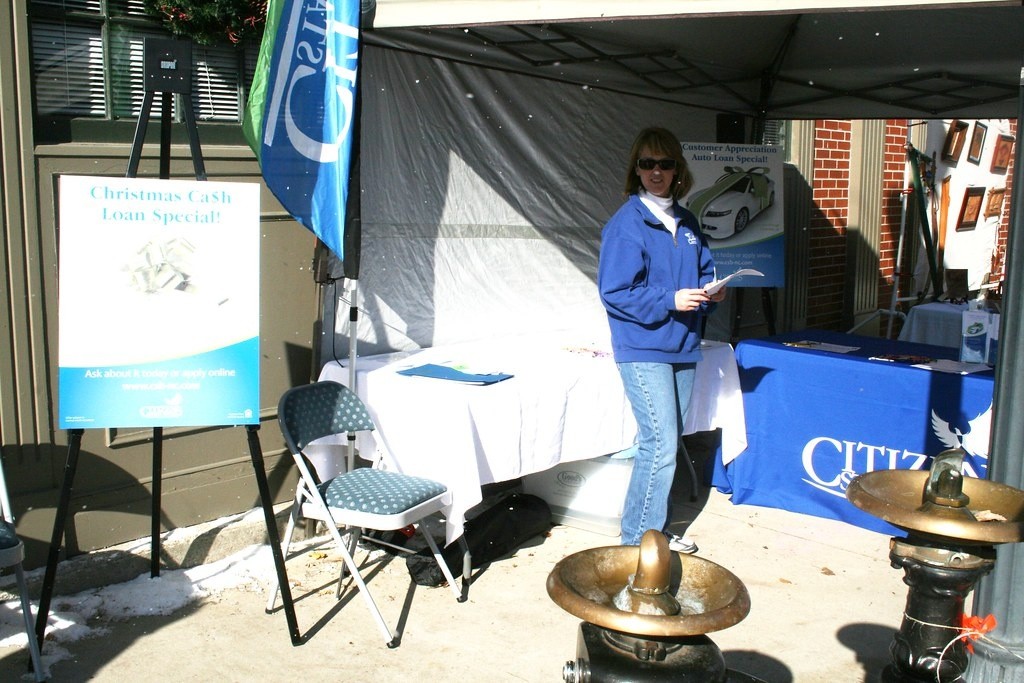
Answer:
[521,448,638,536]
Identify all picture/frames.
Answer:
[990,134,1014,175]
[968,120,988,165]
[941,119,969,164]
[956,186,985,233]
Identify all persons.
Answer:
[599,128,726,553]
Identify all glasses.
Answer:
[637,158,676,171]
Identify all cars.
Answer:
[685,172,776,240]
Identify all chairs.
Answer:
[265,381,469,650]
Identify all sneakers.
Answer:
[664,530,698,554]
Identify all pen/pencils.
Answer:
[782,342,815,347]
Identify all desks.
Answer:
[899,303,1000,366]
[712,328,999,538]
[315,337,748,603]
